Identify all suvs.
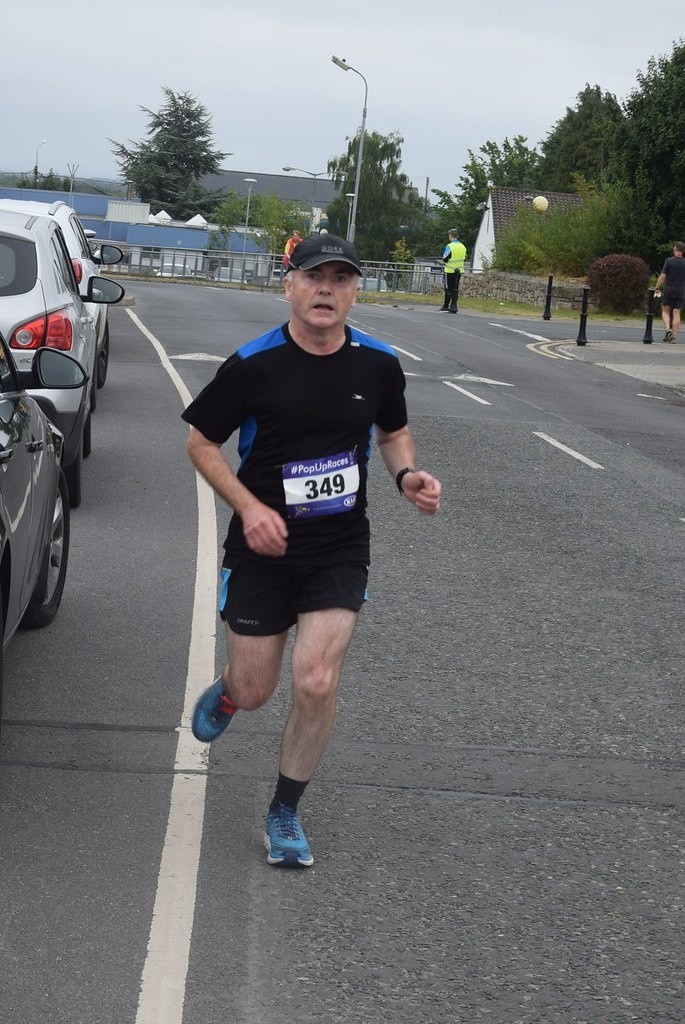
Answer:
[1,207,127,509]
[1,197,124,389]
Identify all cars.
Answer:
[0,332,92,651]
[211,266,249,285]
[360,277,389,293]
[153,263,194,278]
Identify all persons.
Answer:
[655,241,685,344]
[182,234,442,868]
[440,229,467,313]
[283,231,303,271]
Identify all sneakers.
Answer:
[663,330,676,343]
[191,673,240,743]
[263,802,313,868]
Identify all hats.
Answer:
[283,233,365,279]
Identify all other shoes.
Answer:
[438,303,457,314]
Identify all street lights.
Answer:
[66,162,80,207]
[330,55,371,245]
[34,140,47,189]
[239,177,259,291]
[345,193,355,242]
[281,166,349,237]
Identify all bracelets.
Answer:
[656,288,659,290]
[397,468,416,496]
